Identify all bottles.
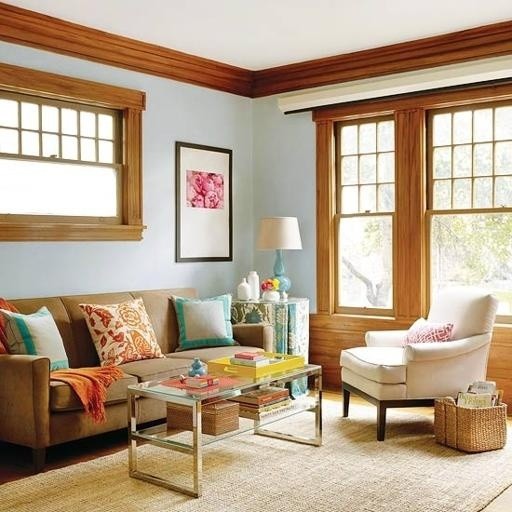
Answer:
[237,271,259,300]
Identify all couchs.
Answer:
[0,285,274,473]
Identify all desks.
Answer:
[231,297,309,395]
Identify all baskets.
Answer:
[432,396,509,454]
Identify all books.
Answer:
[235,351,264,360]
[239,402,294,420]
[238,398,293,412]
[227,386,289,405]
[454,379,505,409]
[225,397,289,407]
[184,375,220,388]
[230,357,270,368]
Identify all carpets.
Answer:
[0,396,512,512]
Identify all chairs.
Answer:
[337,293,499,441]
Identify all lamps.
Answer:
[255,216,302,292]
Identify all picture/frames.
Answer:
[173,140,233,263]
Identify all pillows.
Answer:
[0,293,240,373]
[401,314,455,349]
